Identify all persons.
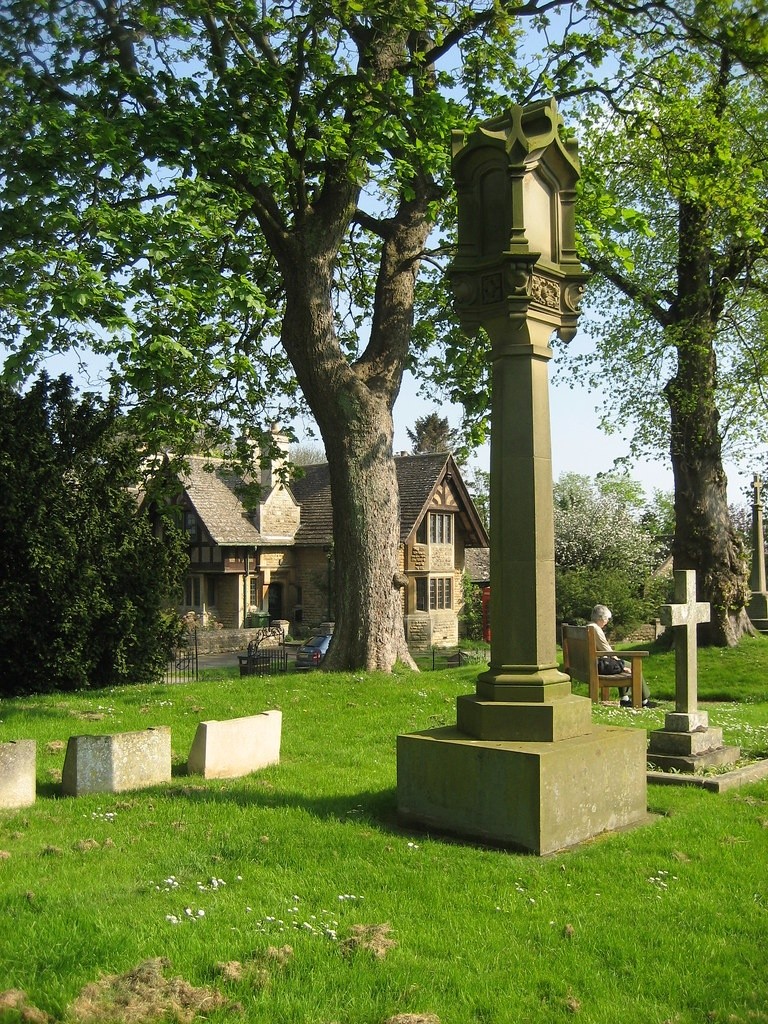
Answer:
[587,604,657,707]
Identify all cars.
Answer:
[295,633,332,668]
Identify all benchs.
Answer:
[561,622,648,708]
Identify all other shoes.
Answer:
[619,700,633,707]
[642,700,659,708]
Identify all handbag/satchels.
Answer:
[598,656,624,675]
